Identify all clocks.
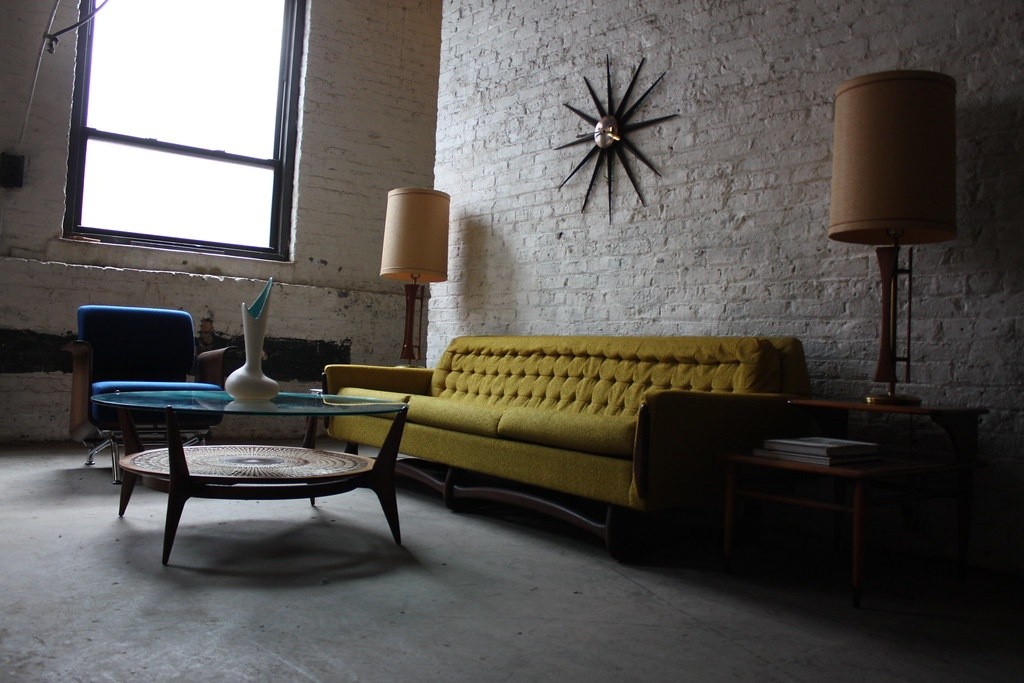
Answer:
[554,54,680,225]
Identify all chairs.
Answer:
[62,304,239,485]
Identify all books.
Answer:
[752,436,884,466]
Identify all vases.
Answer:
[224,276,279,400]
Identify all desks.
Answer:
[718,450,910,607]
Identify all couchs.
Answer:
[319,330,811,567]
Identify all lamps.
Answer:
[379,187,450,367]
[823,70,958,406]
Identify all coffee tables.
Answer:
[92,391,412,566]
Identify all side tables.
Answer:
[780,396,989,598]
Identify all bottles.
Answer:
[225,275,279,402]
